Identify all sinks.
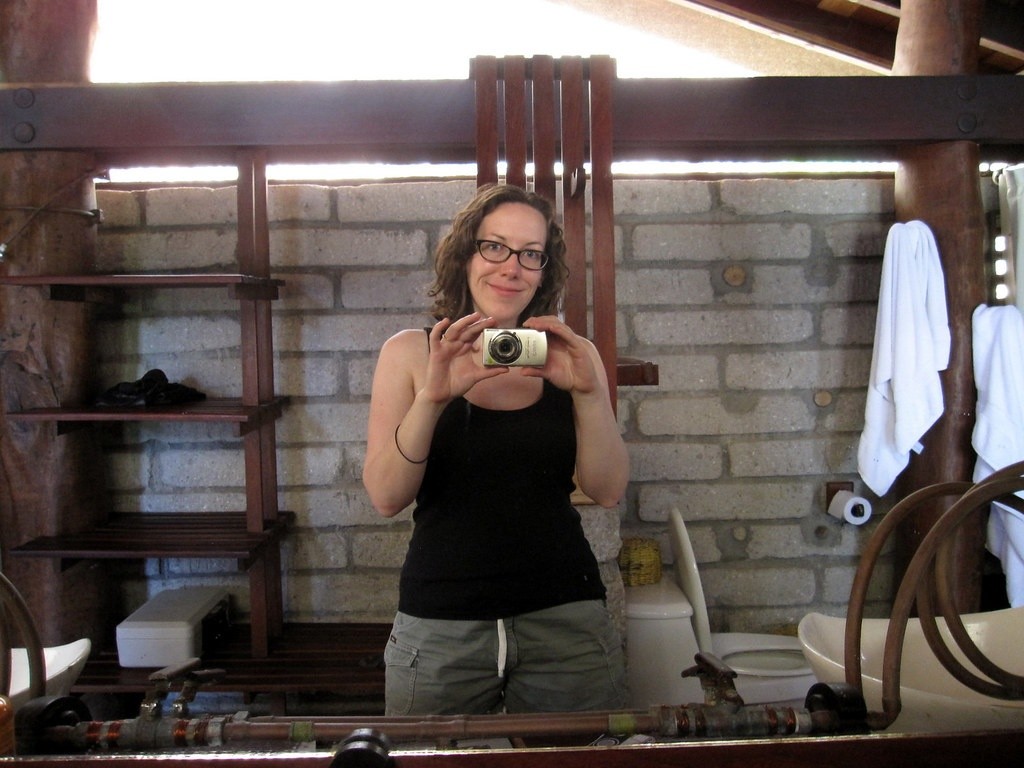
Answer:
[795,596,1024,738]
[8,635,92,725]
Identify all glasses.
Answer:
[474,237,549,271]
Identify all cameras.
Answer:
[481,328,548,367]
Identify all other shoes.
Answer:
[95,370,208,408]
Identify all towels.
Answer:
[971,302,1024,609]
[856,220,954,499]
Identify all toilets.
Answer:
[614,563,820,720]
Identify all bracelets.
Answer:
[394,424,429,464]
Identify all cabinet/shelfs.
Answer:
[5,144,301,695]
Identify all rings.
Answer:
[441,334,455,342]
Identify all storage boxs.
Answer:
[114,586,230,668]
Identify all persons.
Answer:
[360,182,632,716]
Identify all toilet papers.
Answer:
[827,490,873,528]
